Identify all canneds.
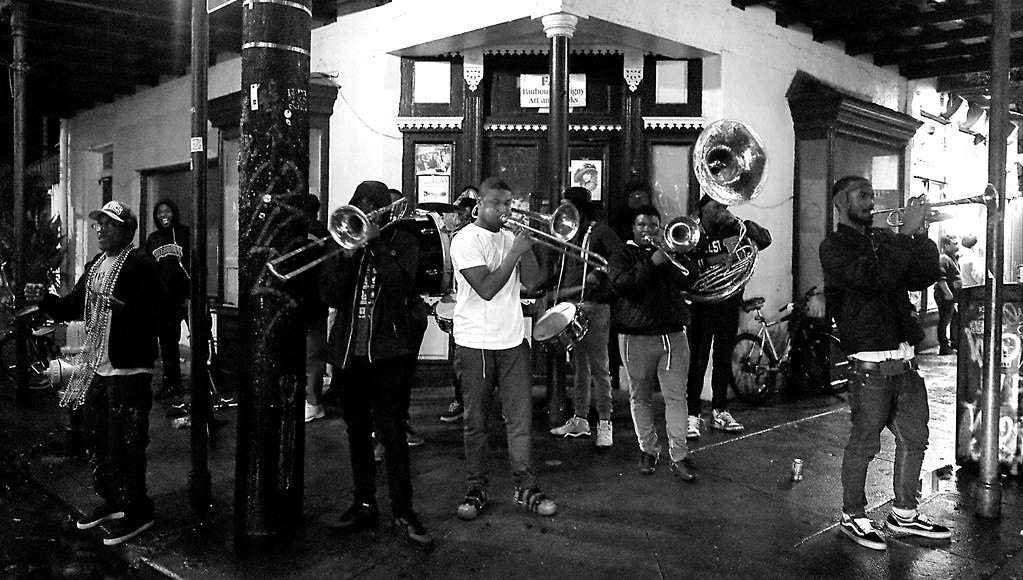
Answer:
[790,459,804,481]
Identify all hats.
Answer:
[88,201,138,230]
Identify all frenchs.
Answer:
[677,118,771,306]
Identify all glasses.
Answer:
[90,222,120,232]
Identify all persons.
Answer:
[817,176,951,550]
[302,193,327,422]
[450,177,557,520]
[530,187,624,448]
[319,180,434,551]
[25,199,176,544]
[934,235,963,355]
[441,187,480,421]
[681,196,772,438]
[958,233,986,286]
[144,196,231,399]
[607,206,698,483]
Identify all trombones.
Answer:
[265,196,409,284]
[643,214,701,278]
[469,201,612,277]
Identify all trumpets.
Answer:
[868,181,1002,229]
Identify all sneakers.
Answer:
[305,399,325,423]
[710,409,745,432]
[596,419,613,448]
[549,416,590,438]
[887,509,951,538]
[441,400,464,421]
[840,514,887,549]
[685,414,704,439]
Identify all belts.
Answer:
[857,362,912,372]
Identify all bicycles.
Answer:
[0,259,63,395]
[732,286,853,402]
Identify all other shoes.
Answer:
[103,510,154,545]
[669,461,698,480]
[636,451,656,474]
[457,489,490,521]
[939,347,957,355]
[332,502,379,531]
[514,489,557,516]
[397,522,434,551]
[373,444,387,462]
[405,425,424,446]
[76,503,126,530]
[158,381,238,428]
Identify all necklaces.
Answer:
[57,242,134,410]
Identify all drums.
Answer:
[530,301,592,358]
[398,207,457,300]
